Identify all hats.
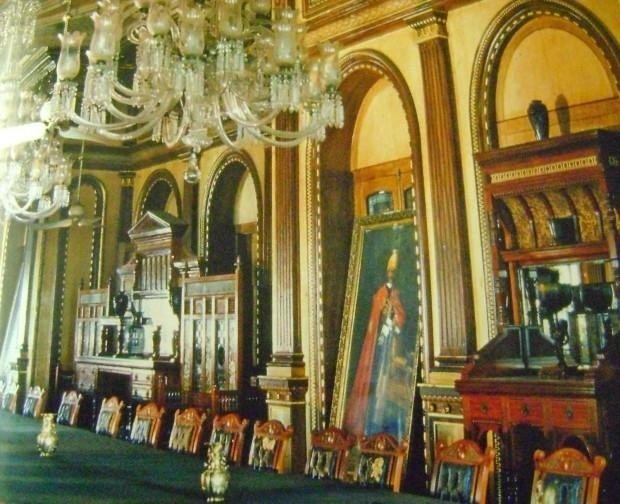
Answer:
[386,253,399,272]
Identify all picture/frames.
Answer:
[327,209,423,481]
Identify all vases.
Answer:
[527,98,550,142]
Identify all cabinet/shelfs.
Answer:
[73,356,180,404]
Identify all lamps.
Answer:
[520,270,615,368]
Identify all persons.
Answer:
[347,250,406,433]
